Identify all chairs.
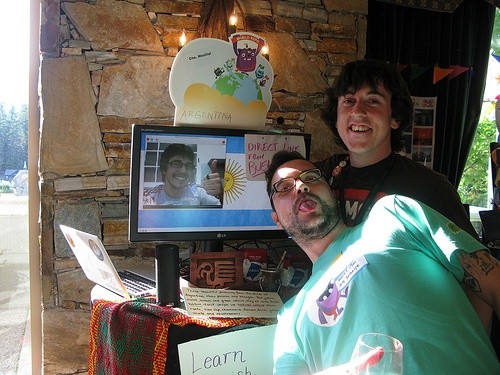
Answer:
[480,211,500,243]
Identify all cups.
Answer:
[260,267,284,296]
[155,244,181,308]
[347,333,403,375]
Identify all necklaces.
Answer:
[338,152,398,228]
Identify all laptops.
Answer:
[59,224,156,300]
[480,209,500,247]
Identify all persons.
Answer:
[264,150,500,375]
[154,143,223,206]
[314,58,494,338]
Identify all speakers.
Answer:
[155,244,179,307]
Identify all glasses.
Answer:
[270,168,329,204]
[168,160,195,172]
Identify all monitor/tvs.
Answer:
[128,124,311,252]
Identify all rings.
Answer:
[206,174,210,179]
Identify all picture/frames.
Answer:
[191,253,243,290]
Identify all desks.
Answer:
[90,268,267,375]
